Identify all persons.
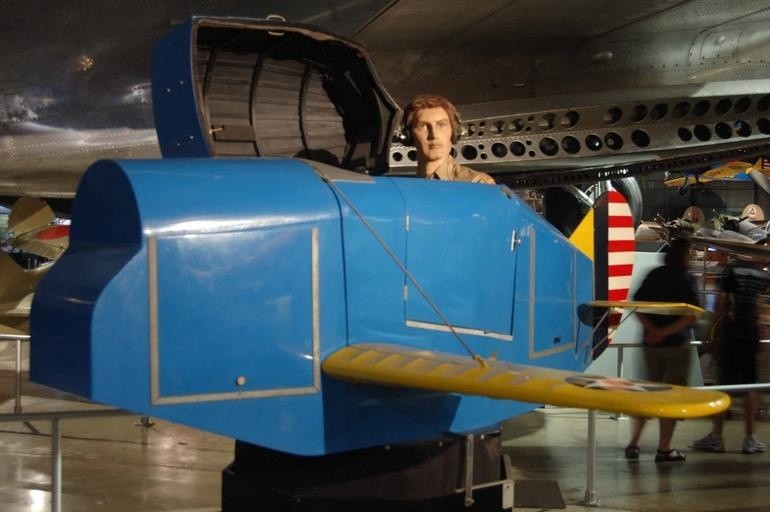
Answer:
[623,228,703,465]
[401,91,498,186]
[691,246,767,454]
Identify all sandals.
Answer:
[654,447,686,462]
[624,443,641,458]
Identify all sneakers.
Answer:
[692,433,725,453]
[741,434,768,454]
